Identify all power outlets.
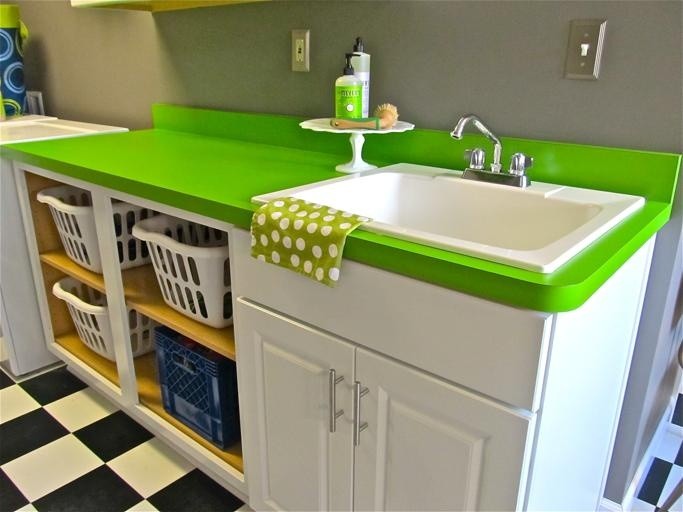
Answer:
[291,28,310,73]
[561,19,603,80]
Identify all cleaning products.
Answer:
[345,36,371,118]
[333,52,364,120]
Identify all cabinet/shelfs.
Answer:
[231,292,540,512]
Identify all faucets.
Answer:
[450,113,502,173]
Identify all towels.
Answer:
[249,196,374,289]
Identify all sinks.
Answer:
[250,163,646,275]
